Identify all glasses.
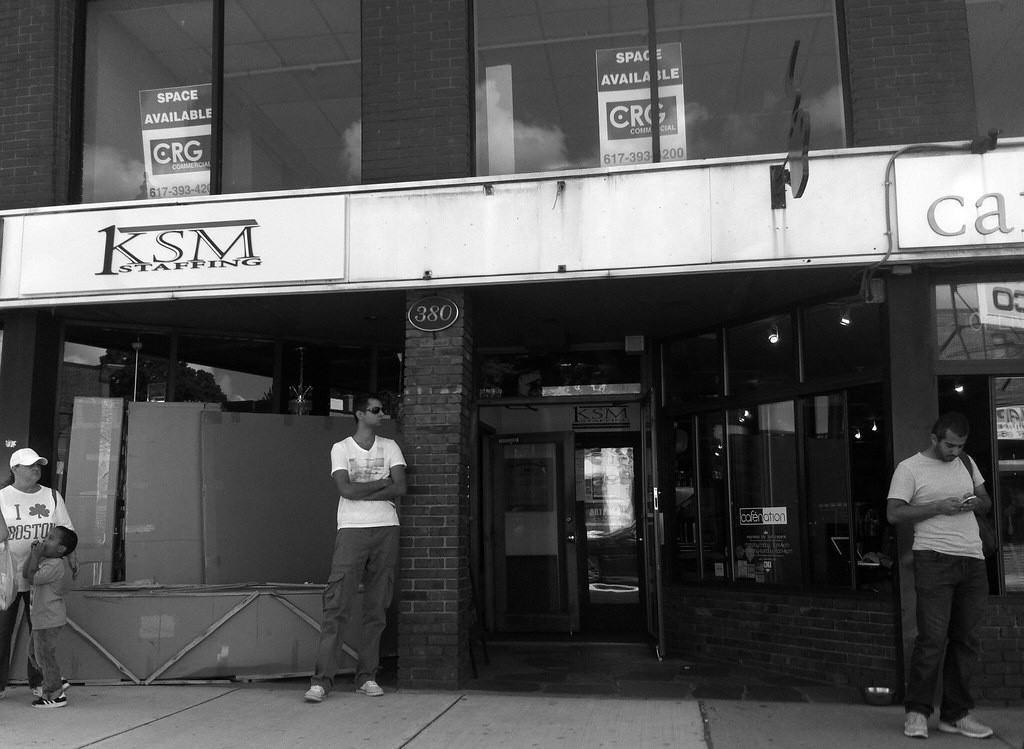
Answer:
[366,407,386,414]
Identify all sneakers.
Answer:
[355,680,385,697]
[31,675,71,709]
[937,713,994,739]
[903,711,929,739]
[0,687,7,699]
[304,683,328,703]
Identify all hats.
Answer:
[9,447,49,470]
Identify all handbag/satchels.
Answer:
[0,538,18,610]
[973,512,1000,559]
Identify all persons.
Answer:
[304,392,408,702]
[23,526,78,709]
[0,448,80,698]
[886,412,994,739]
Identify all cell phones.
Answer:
[962,495,977,504]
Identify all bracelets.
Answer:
[67,555,78,559]
